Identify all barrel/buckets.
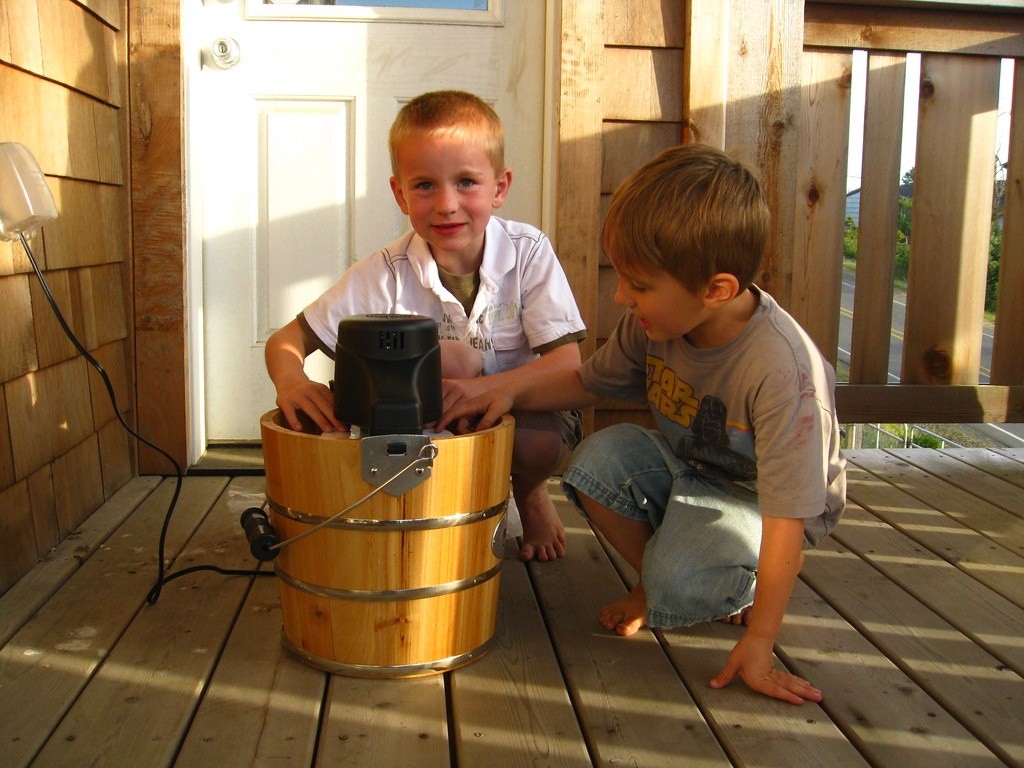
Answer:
[259,406,515,678]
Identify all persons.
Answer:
[265,90,587,561]
[435,143,848,706]
